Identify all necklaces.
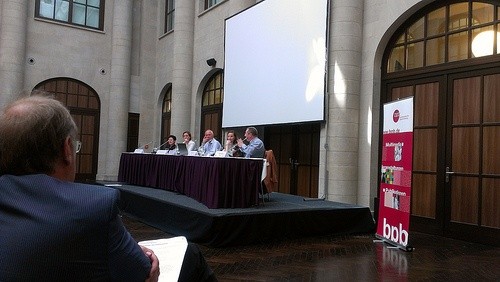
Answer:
[182,131,195,151]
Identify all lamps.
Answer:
[206,57,217,69]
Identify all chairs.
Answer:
[260,149,273,201]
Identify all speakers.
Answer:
[207,58,216,66]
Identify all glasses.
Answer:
[74,140,81,153]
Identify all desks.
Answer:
[117,151,265,209]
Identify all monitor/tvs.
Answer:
[177,143,188,155]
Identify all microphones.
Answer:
[157,141,169,149]
[234,138,247,147]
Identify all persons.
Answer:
[0,97,160,282]
[201,130,221,154]
[223,127,264,157]
[165,135,177,150]
